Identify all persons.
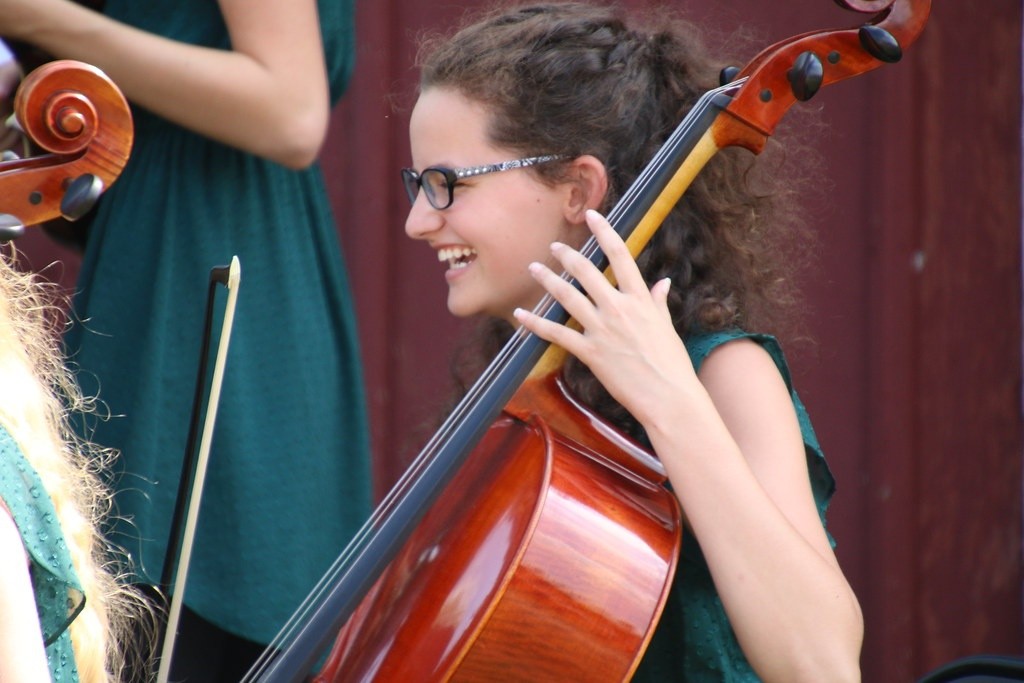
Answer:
[398,2,870,681]
[0,235,114,683]
[0,0,372,619]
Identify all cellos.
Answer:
[235,0,935,683]
[0,59,133,240]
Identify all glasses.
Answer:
[400,153,576,210]
